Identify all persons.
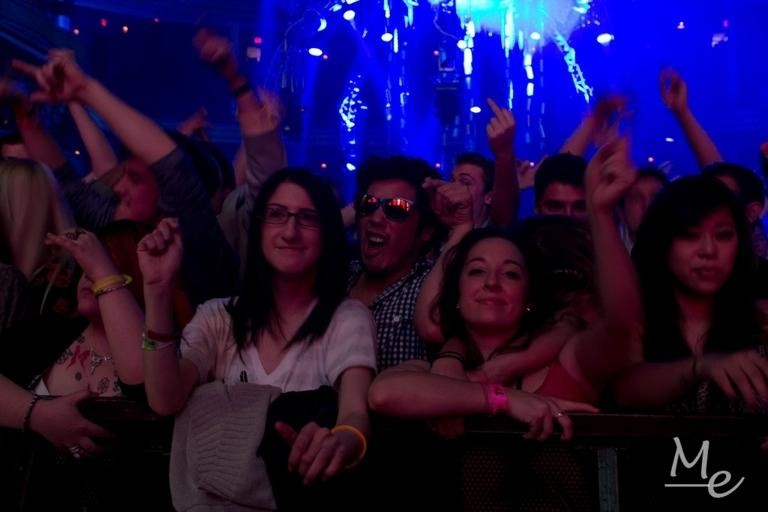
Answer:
[0,30,766,512]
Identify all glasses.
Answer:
[358,193,417,224]
[263,206,321,228]
